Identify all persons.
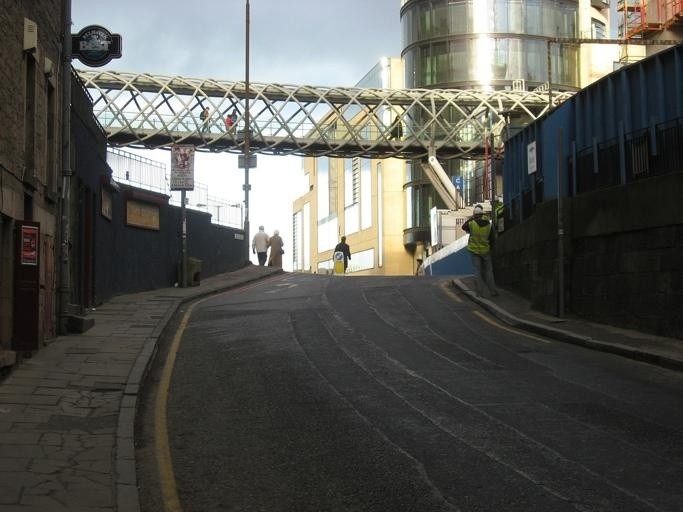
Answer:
[462,206,500,299]
[388,115,403,140]
[252,226,270,267]
[267,230,284,268]
[333,236,351,273]
[199,107,211,133]
[227,114,232,131]
[230,108,239,134]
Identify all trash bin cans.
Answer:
[176,256,202,287]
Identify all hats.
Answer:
[474,207,482,215]
